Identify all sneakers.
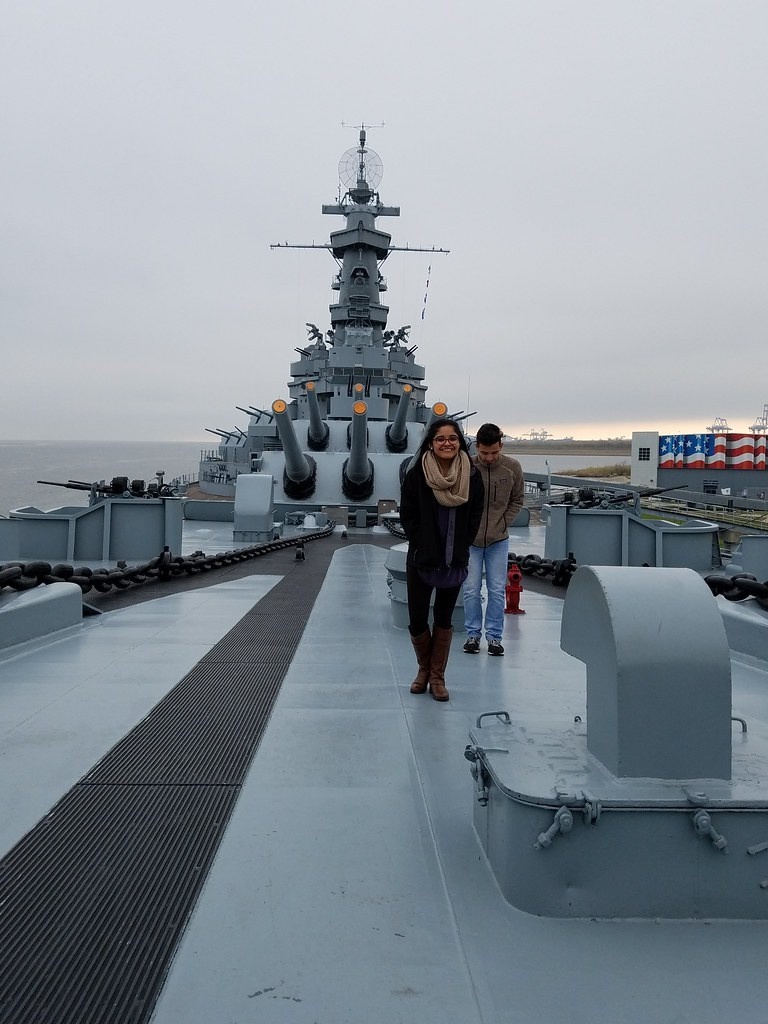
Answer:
[488,640,504,655]
[462,637,480,653]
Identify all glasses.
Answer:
[433,436,459,446]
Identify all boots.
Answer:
[429,623,455,700]
[408,623,431,694]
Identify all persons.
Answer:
[400,420,485,702]
[462,424,524,655]
